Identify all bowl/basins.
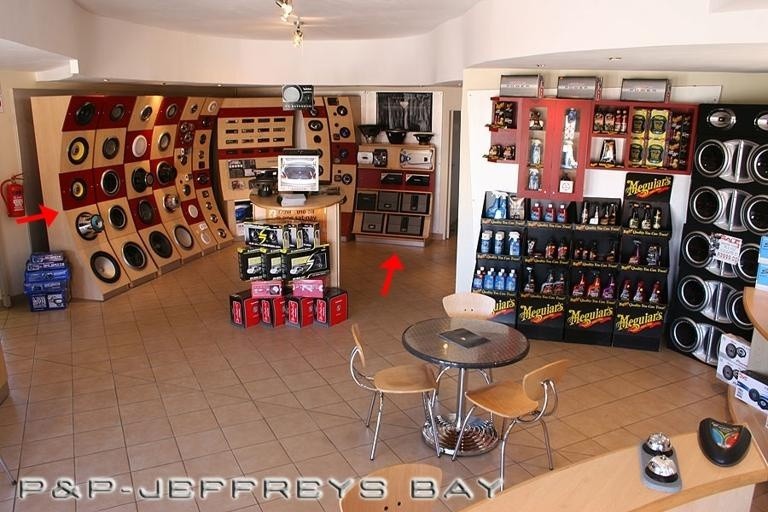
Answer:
[386,129,407,145]
[413,134,434,144]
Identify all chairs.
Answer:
[435,291,498,388]
[338,461,444,511]
[449,359,575,492]
[348,323,445,462]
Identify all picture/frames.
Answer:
[276,154,321,193]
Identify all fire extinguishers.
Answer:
[0,174,25,217]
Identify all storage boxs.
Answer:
[228,219,349,329]
[23,249,72,313]
[712,235,768,412]
[498,73,673,103]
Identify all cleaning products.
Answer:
[488,102,629,192]
[473,190,664,304]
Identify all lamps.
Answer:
[272,1,310,54]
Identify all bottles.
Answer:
[544,236,661,268]
[494,102,514,126]
[592,109,627,133]
[529,202,663,229]
[474,266,662,305]
[482,230,505,254]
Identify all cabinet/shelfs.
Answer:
[515,194,576,343]
[568,195,622,346]
[589,102,698,175]
[470,189,527,333]
[490,96,590,198]
[352,141,434,246]
[613,198,676,353]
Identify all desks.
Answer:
[402,317,533,454]
[246,192,347,297]
